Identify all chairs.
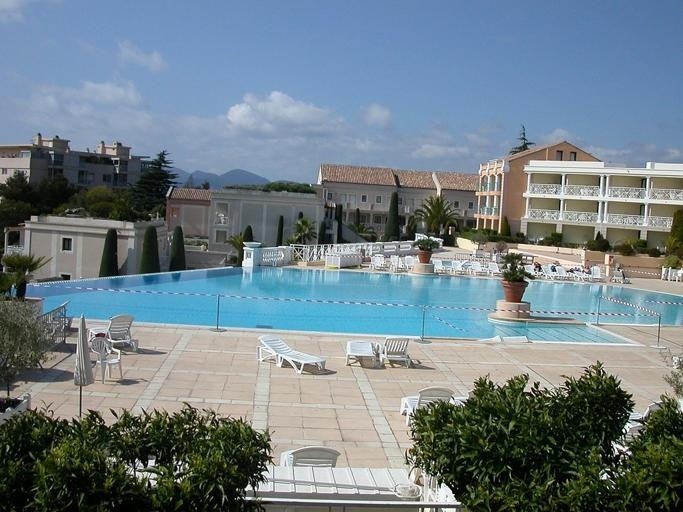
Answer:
[278,444,341,468]
[85,314,139,384]
[256,335,416,374]
[371,253,633,284]
[399,386,680,450]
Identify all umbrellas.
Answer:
[72,314,94,417]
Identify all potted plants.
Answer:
[412,237,440,263]
[498,252,529,303]
